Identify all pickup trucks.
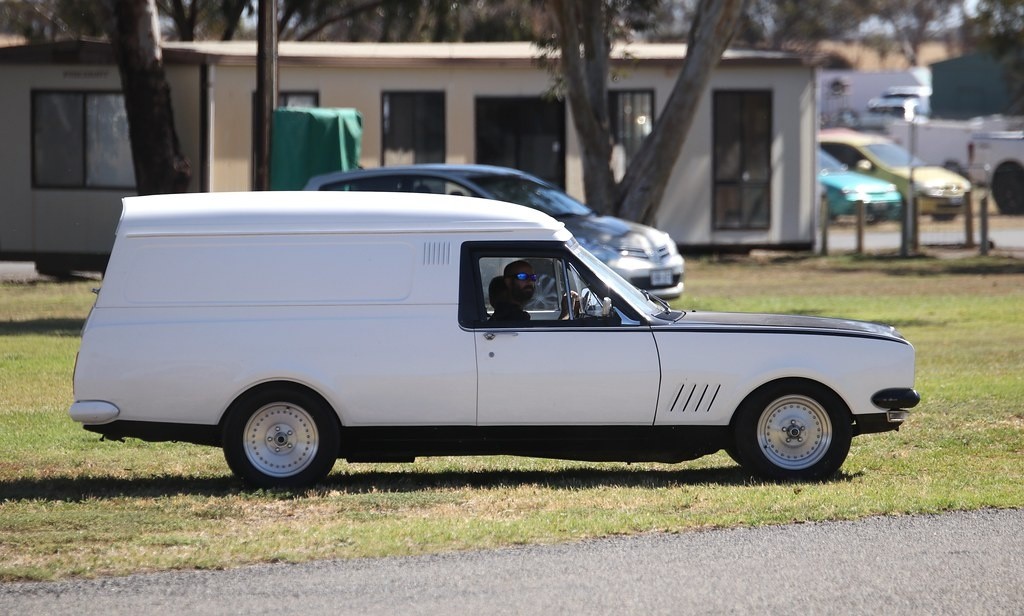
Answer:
[959,114,1024,217]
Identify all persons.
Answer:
[486,261,580,321]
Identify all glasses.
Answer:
[506,272,537,282]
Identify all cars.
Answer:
[818,127,972,220]
[301,163,685,310]
[64,188,923,496]
[817,147,903,226]
